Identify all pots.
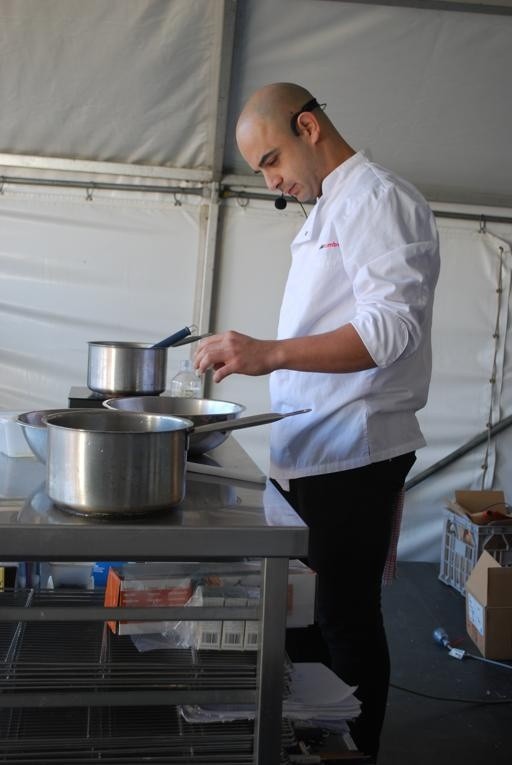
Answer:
[83,332,215,396]
[40,407,314,518]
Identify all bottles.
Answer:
[168,357,205,399]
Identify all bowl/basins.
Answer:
[14,393,247,471]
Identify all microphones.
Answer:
[274,192,288,210]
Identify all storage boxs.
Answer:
[447,490,510,662]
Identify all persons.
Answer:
[190,82,440,764]
